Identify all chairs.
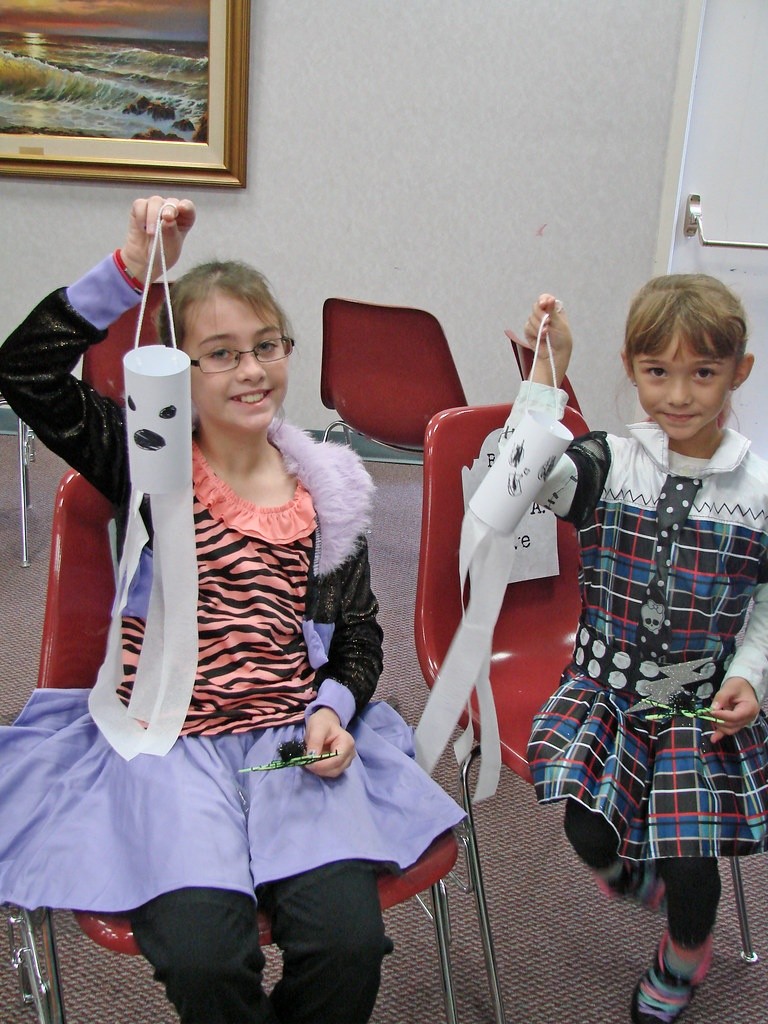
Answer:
[416,403,757,1024]
[7,468,459,1024]
[321,298,467,465]
[505,328,583,417]
[19,282,172,567]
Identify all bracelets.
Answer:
[116,248,151,291]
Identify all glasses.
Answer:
[190,337,295,373]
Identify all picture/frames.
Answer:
[0,0,251,188]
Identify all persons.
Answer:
[520,272,768,1024]
[0,193,388,1024]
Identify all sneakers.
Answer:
[594,863,667,913]
[631,928,718,1023]
